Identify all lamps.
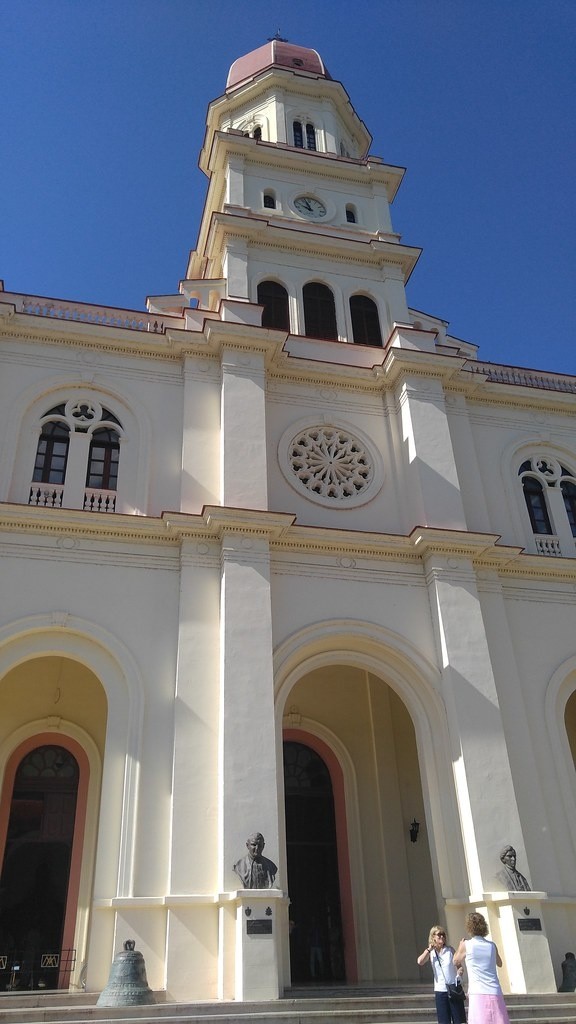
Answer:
[410,818,420,842]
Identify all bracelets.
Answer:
[427,948,430,953]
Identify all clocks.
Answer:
[295,197,326,220]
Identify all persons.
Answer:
[416,926,467,1024]
[495,844,531,892]
[233,832,277,889]
[452,912,511,1024]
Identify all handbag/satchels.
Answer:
[446,984,467,1000]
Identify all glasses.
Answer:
[433,932,446,937]
